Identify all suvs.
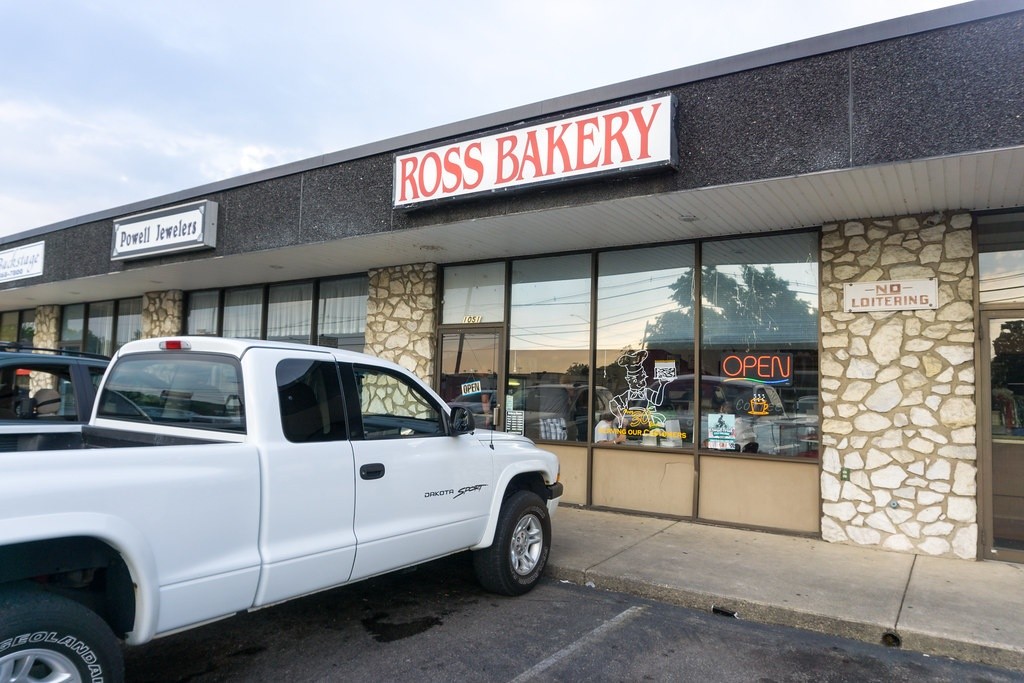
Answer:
[509,382,618,441]
[0,340,169,425]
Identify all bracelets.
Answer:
[613,440,617,444]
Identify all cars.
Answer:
[612,373,819,460]
[1006,382,1024,403]
[446,388,499,430]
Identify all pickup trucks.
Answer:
[0,335,566,683]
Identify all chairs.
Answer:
[35,389,61,418]
[278,381,346,441]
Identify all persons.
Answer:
[524,358,557,439]
[593,407,626,445]
[574,366,599,441]
[539,357,576,440]
[474,392,493,428]
